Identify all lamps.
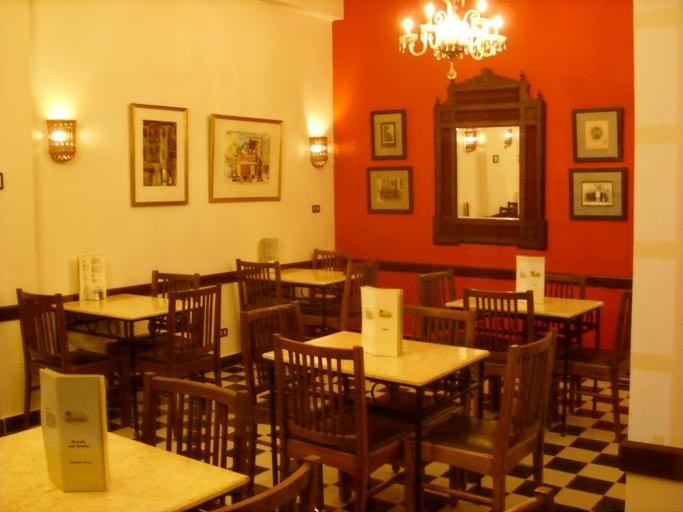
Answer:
[462,126,479,153]
[392,1,508,82]
[45,117,77,163]
[498,127,514,149]
[309,133,329,171]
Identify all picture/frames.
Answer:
[369,108,405,159]
[126,100,190,208]
[366,165,413,214]
[207,113,284,205]
[572,107,623,162]
[567,165,625,221]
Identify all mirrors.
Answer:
[430,64,552,249]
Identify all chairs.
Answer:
[487,200,517,219]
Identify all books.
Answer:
[359,285,403,357]
[37,367,111,495]
[516,254,547,302]
[79,255,105,299]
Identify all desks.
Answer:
[1,244,632,512]
